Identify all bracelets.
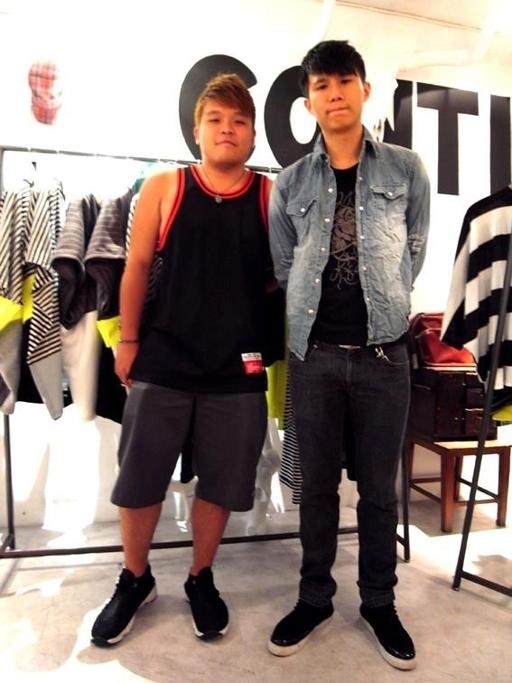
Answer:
[118,338,140,344]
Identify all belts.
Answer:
[307,339,408,358]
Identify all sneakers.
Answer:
[358,602,417,671]
[91,564,158,648]
[265,598,334,657]
[182,565,229,642]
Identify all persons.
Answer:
[90,70,275,647]
[267,39,431,672]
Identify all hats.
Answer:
[27,60,67,126]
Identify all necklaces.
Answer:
[201,165,246,204]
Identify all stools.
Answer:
[402,427,512,533]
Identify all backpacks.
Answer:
[409,308,479,372]
